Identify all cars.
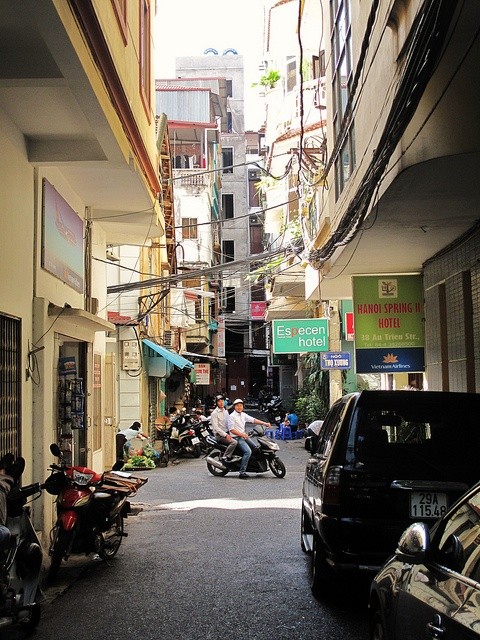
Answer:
[369,483,480,639]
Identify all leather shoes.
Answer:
[239,473,249,479]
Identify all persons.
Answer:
[210,395,238,465]
[167,406,177,417]
[111,421,150,470]
[306,419,323,446]
[194,410,207,423]
[285,410,298,433]
[0,451,26,531]
[228,399,271,480]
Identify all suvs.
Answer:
[301,390,479,598]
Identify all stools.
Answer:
[281,426,292,440]
[264,430,272,438]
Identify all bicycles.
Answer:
[158,424,172,467]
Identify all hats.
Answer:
[216,395,225,400]
[233,399,243,404]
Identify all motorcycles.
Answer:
[47,444,147,584]
[260,394,286,425]
[181,414,209,455]
[0,481,54,633]
[168,417,200,458]
[202,424,286,478]
